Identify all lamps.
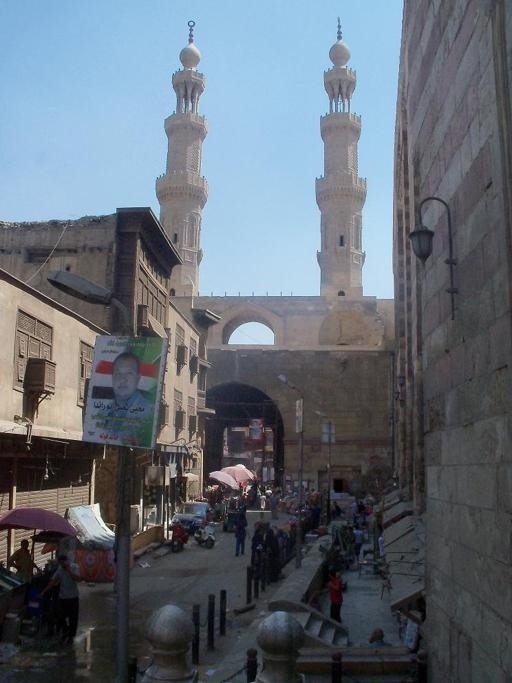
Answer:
[404,192,463,324]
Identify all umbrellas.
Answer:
[208,463,255,490]
[0,507,77,578]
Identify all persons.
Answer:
[368,628,393,649]
[40,555,82,645]
[9,539,40,605]
[102,350,153,418]
[205,476,387,623]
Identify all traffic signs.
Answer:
[295,398,303,433]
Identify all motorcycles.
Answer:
[193,519,216,548]
[171,518,190,553]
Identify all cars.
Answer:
[171,500,217,535]
